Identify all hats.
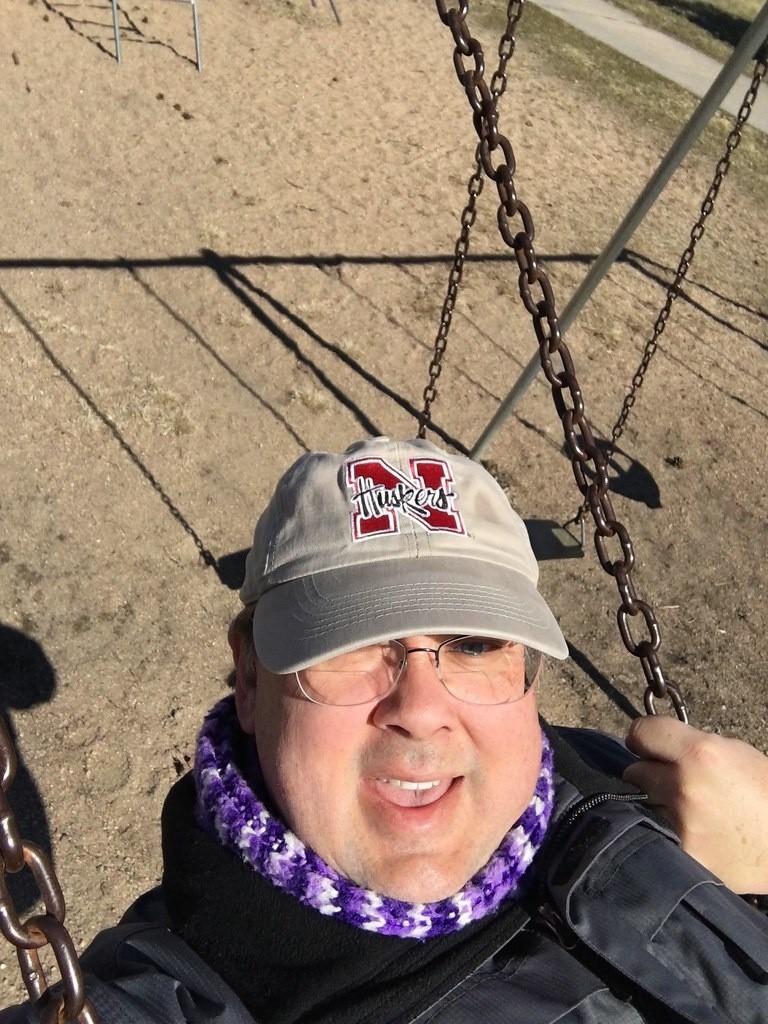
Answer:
[242,435,568,673]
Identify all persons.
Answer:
[1,436,768,1023]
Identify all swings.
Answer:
[419,0,768,563]
[0,0,691,1024]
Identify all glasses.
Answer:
[309,636,543,716]
